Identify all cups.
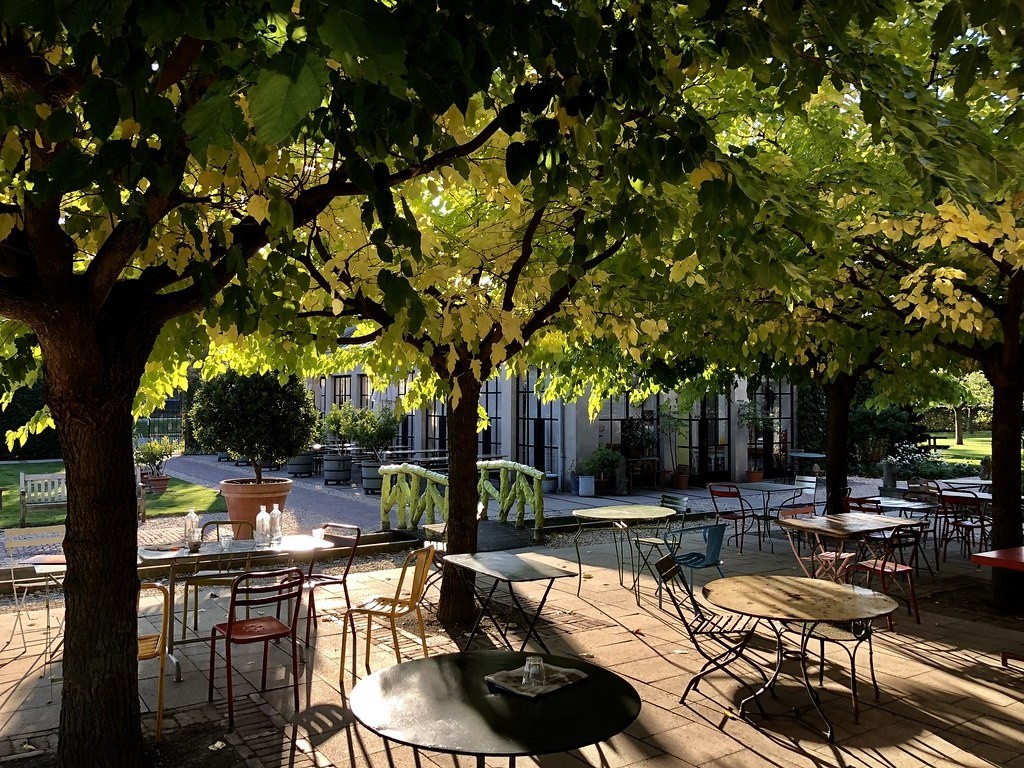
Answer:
[189,528,202,540]
[253,530,256,543]
[522,656,546,686]
[187,541,206,552]
[220,537,232,549]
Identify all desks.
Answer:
[703,574,898,744]
[138,535,333,682]
[308,443,508,464]
[870,499,942,551]
[349,650,641,768]
[774,513,930,584]
[442,552,579,655]
[708,481,814,554]
[572,505,676,606]
[789,452,827,476]
[924,489,994,543]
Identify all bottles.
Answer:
[269,504,282,543]
[184,508,199,548]
[256,505,270,546]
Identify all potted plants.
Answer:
[674,465,689,490]
[622,419,658,459]
[191,371,403,545]
[136,437,178,495]
[580,446,622,495]
[738,400,782,482]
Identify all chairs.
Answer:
[40,480,1024,768]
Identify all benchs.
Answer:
[314,456,506,495]
[19,467,145,530]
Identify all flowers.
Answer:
[567,457,583,474]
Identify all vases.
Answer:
[570,474,579,496]
